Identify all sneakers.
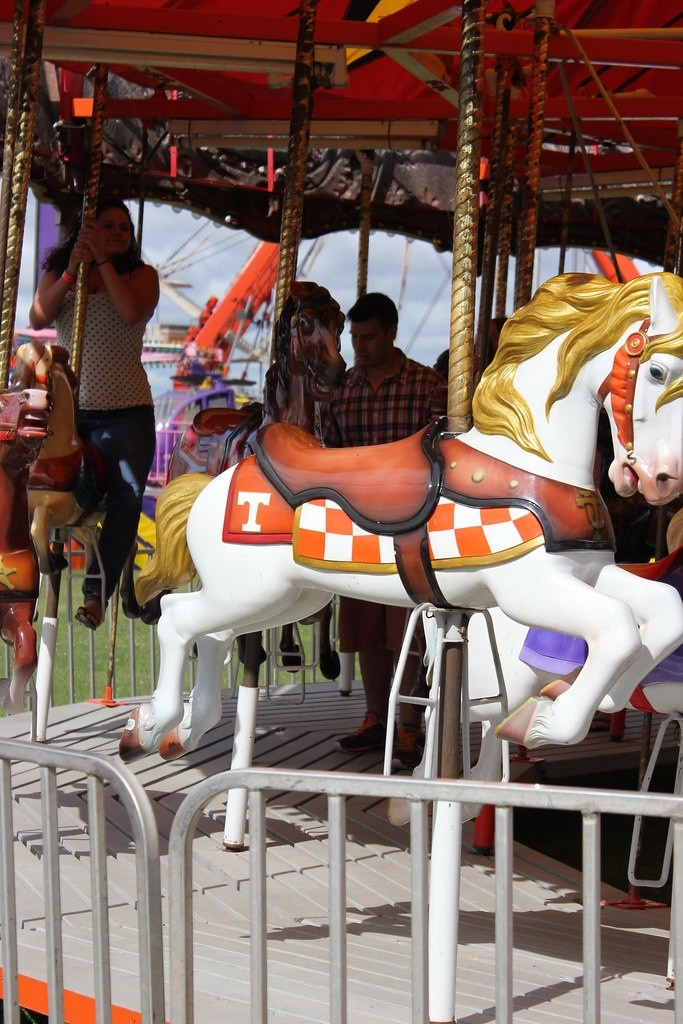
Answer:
[393,722,425,769]
[335,711,397,752]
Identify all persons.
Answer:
[317,291,450,770]
[28,198,160,630]
[594,409,650,566]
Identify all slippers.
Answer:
[76,606,100,630]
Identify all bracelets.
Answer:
[97,259,109,266]
[61,270,76,283]
